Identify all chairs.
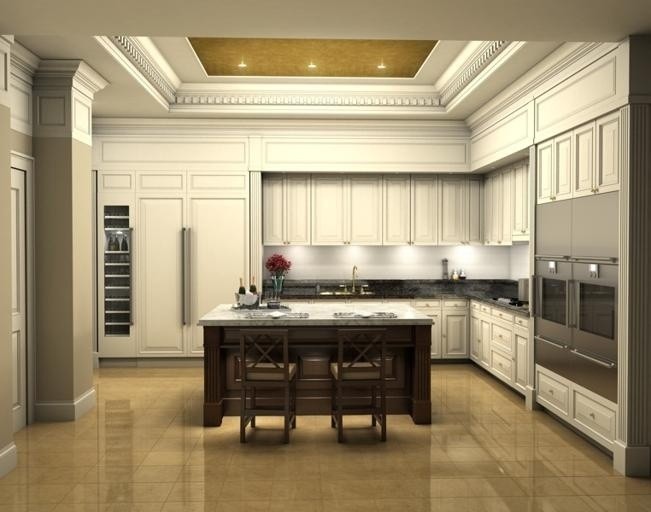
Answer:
[240,329,297,443]
[329,329,385,443]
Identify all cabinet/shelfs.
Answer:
[383,174,438,246]
[534,364,617,451]
[537,128,573,205]
[438,174,484,246]
[262,173,311,246]
[311,174,383,246]
[413,300,528,398]
[573,107,620,199]
[484,161,513,246]
[97,170,137,367]
[135,171,250,369]
[513,155,529,241]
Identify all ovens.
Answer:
[534,191,619,404]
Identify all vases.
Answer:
[271,275,284,300]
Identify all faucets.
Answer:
[352,265,360,292]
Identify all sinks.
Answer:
[320,291,353,296]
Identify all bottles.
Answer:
[238,276,256,294]
[108,233,128,251]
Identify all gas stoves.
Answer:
[491,295,530,312]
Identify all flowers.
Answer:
[265,254,291,290]
